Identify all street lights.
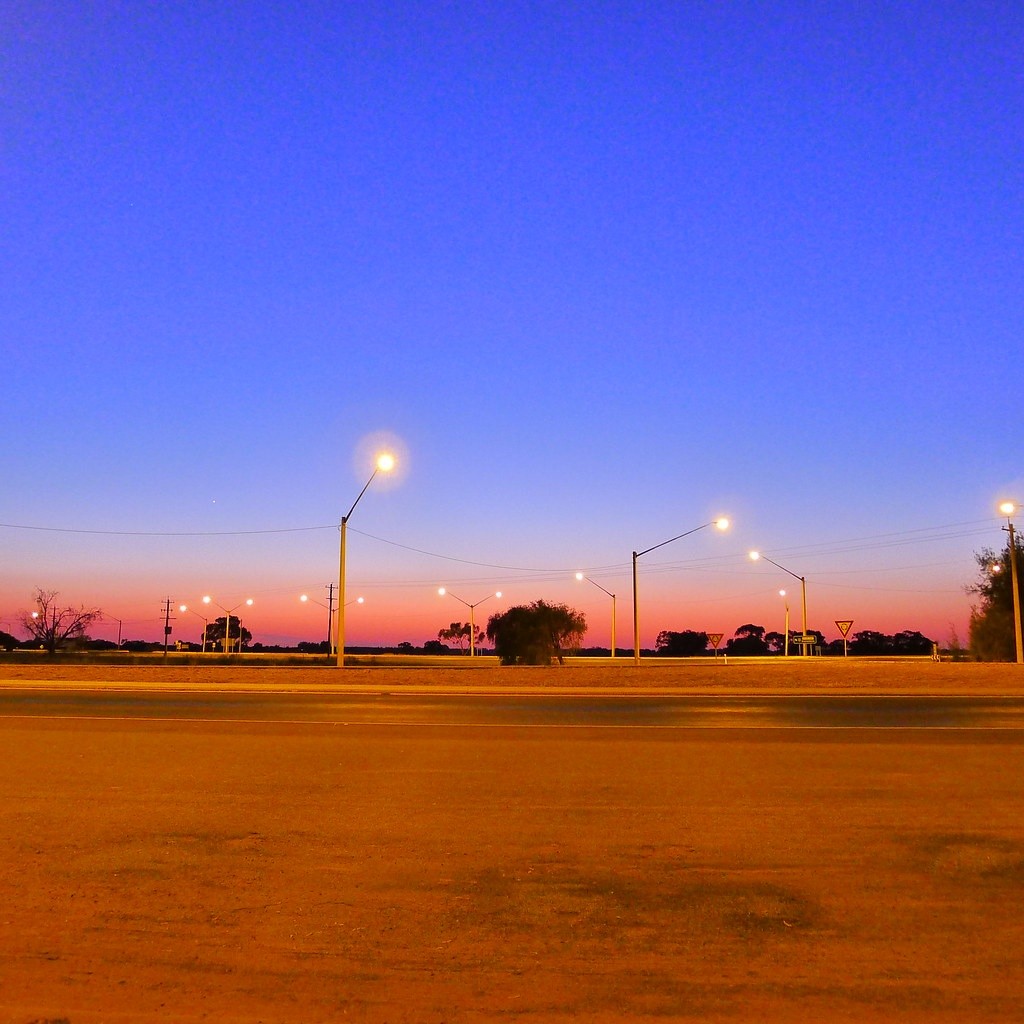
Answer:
[991,503,1024,664]
[96,610,121,650]
[749,552,808,656]
[632,518,729,665]
[337,453,395,662]
[439,588,502,657]
[577,574,616,658]
[202,597,254,653]
[301,595,363,655]
[179,606,207,653]
[779,591,789,656]
